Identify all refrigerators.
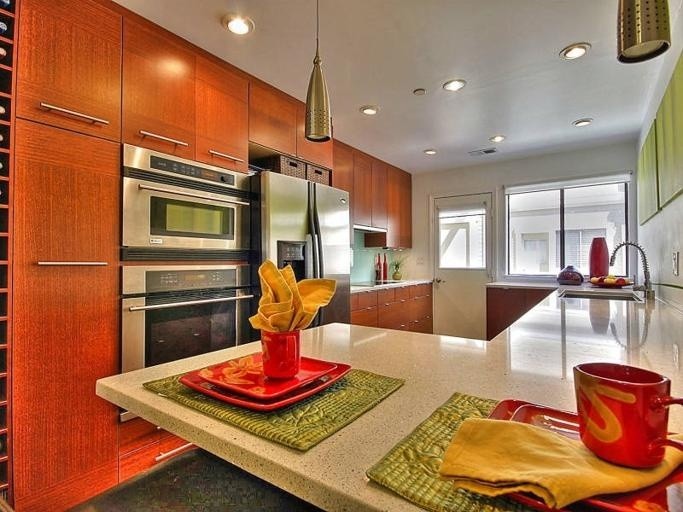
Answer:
[247,169,351,339]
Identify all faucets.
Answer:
[610,241,655,300]
[611,302,654,352]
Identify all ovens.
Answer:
[116,144,252,424]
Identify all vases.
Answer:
[557,264,584,285]
[587,297,610,335]
[588,237,610,285]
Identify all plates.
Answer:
[588,280,634,289]
[178,352,351,411]
[484,399,682,511]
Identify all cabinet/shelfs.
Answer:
[332,139,354,251]
[253,82,333,185]
[486,286,553,343]
[119,412,205,483]
[351,147,388,233]
[122,12,251,169]
[5,2,122,511]
[349,282,433,351]
[364,163,412,247]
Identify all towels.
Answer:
[247,259,338,342]
[437,402,680,512]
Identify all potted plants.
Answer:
[390,257,407,280]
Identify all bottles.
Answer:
[375,252,381,285]
[382,253,387,284]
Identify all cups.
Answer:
[261,332,301,379]
[572,361,682,472]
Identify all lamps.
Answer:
[301,1,335,143]
[612,2,673,65]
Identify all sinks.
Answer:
[558,290,643,302]
